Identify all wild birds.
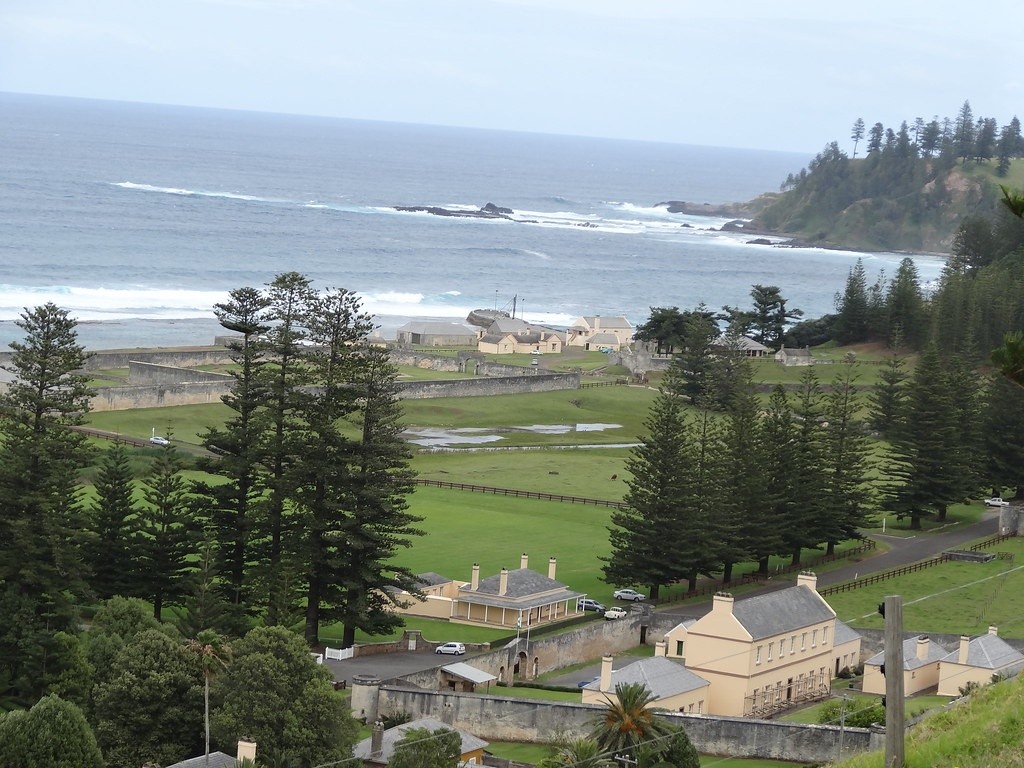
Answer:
[610,473,618,481]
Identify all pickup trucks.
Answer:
[985,498,1009,508]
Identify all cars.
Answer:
[605,606,627,620]
[435,642,466,656]
[602,348,615,353]
[530,350,543,356]
[150,436,171,445]
[579,599,607,613]
[614,589,647,602]
